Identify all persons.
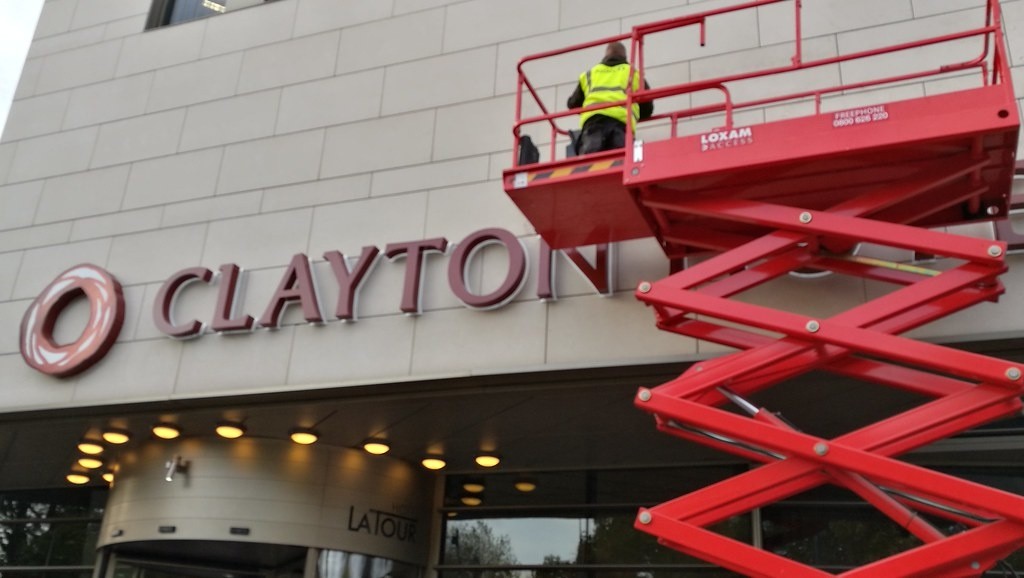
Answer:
[567,42,653,156]
